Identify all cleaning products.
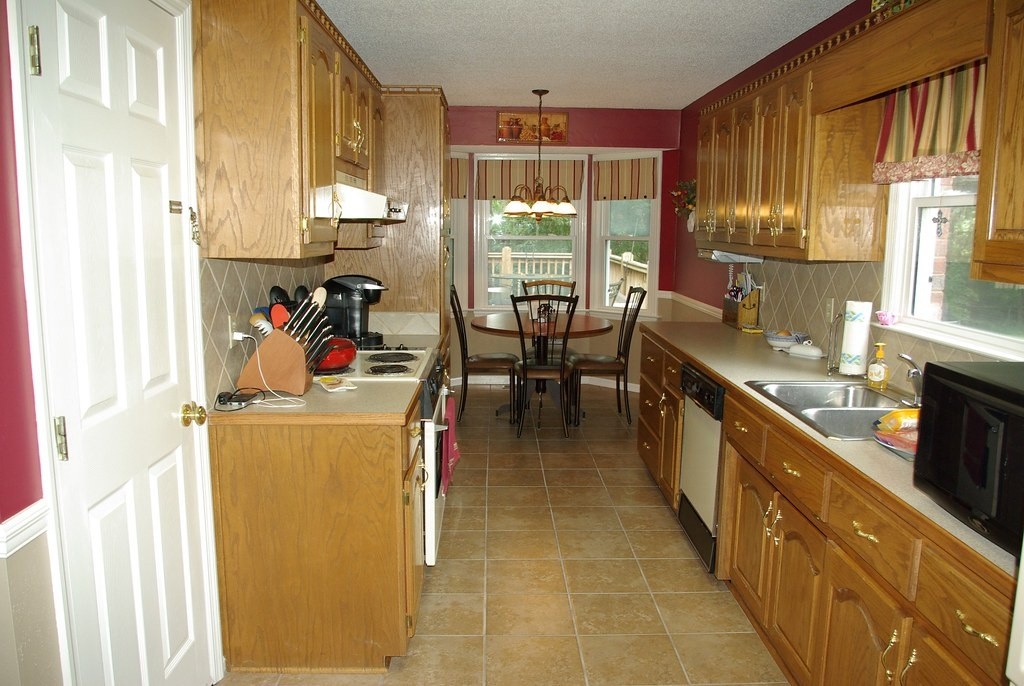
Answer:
[866,343,890,389]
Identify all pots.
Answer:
[307,338,357,368]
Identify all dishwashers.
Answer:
[672,364,744,575]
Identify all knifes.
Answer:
[281,289,335,374]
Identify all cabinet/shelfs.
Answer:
[696,113,715,250]
[192,0,340,263]
[636,332,666,485]
[368,82,388,248]
[323,79,452,393]
[750,72,888,262]
[973,1,1024,284]
[723,395,832,686]
[711,96,759,253]
[331,46,370,179]
[658,352,686,512]
[214,379,427,673]
[820,475,1013,686]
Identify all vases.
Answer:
[686,207,696,234]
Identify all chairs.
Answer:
[567,285,648,427]
[608,278,625,306]
[510,293,580,439]
[447,284,522,427]
[519,280,578,407]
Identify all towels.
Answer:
[442,397,460,493]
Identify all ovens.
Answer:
[409,379,456,573]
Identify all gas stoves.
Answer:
[304,335,446,398]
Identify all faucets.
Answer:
[897,353,924,407]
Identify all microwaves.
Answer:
[909,357,1024,560]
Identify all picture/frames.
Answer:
[495,109,570,145]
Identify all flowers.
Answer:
[667,177,698,222]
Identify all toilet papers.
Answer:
[839,301,873,375]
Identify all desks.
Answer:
[471,311,616,428]
[491,273,572,306]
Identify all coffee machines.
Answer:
[320,269,384,345]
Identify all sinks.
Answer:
[745,379,917,410]
[797,403,924,440]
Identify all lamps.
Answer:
[502,88,579,224]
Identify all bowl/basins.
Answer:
[763,329,809,351]
